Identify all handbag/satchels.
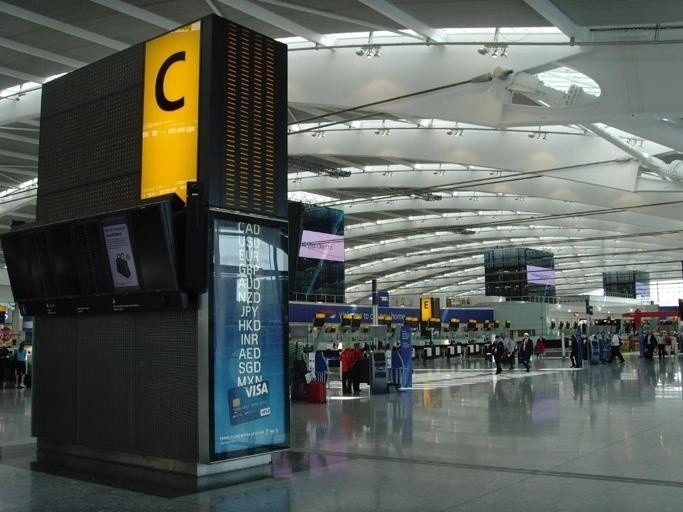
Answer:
[295,359,307,372]
[491,349,529,363]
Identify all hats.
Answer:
[344,342,360,349]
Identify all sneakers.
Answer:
[569,356,672,370]
[13,383,28,389]
[495,367,530,375]
[341,388,363,394]
[494,331,530,340]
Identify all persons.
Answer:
[287,330,308,403]
[567,333,578,369]
[489,335,505,374]
[519,331,533,370]
[338,342,359,394]
[504,336,517,370]
[12,341,28,389]
[601,330,683,363]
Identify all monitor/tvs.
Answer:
[551,321,578,329]
[374,352,385,361]
[314,317,511,327]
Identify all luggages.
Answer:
[644,351,649,360]
[303,383,326,403]
